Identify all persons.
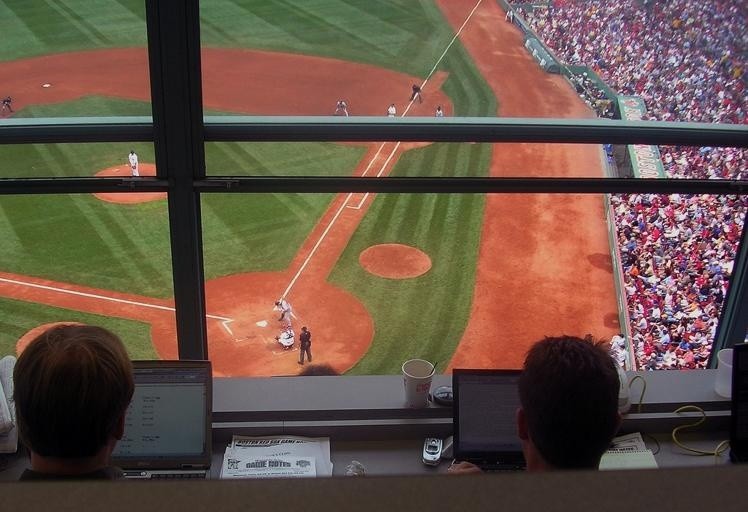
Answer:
[296,327,312,366]
[276,324,296,351]
[504,0,747,370]
[433,104,442,116]
[407,83,423,104]
[333,99,348,116]
[385,102,396,118]
[11,321,132,480]
[1,94,15,114]
[446,334,623,476]
[128,148,139,177]
[273,298,299,329]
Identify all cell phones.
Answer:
[422,437,443,466]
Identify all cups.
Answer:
[713,349,733,398]
[401,358,435,408]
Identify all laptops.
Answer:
[729,343,748,464]
[109,360,213,481]
[452,368,529,473]
